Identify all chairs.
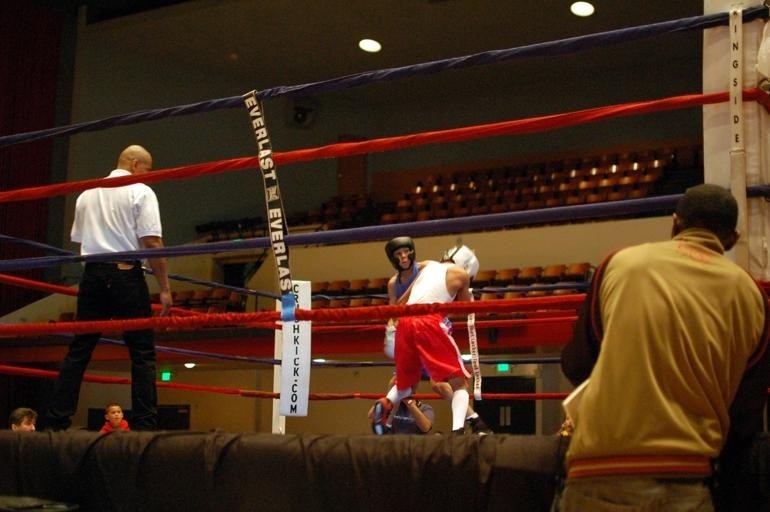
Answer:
[379,148,673,229]
[212,146,370,228]
[305,261,594,323]
[47,284,250,324]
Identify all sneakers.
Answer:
[472,418,492,436]
[372,398,393,434]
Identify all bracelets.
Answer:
[405,399,418,408]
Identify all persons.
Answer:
[546,183,770,511]
[44,144,175,432]
[7,408,39,433]
[381,235,493,436]
[368,242,480,441]
[557,414,572,435]
[367,371,436,435]
[99,402,132,432]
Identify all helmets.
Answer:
[445,244,479,279]
[385,236,415,270]
